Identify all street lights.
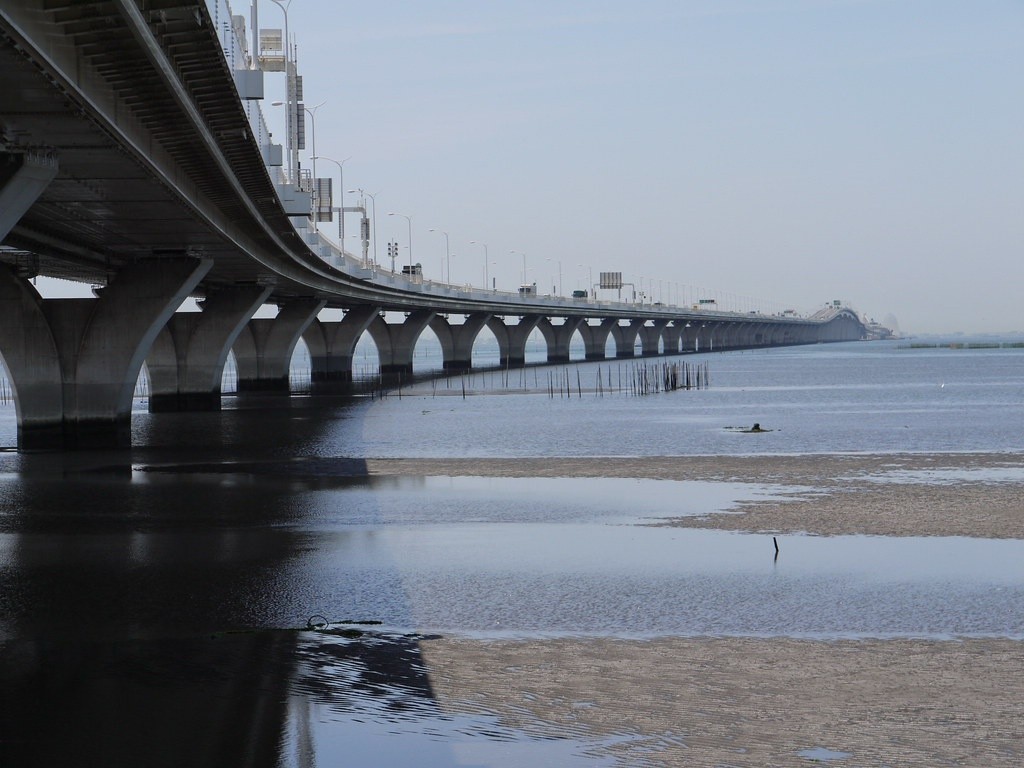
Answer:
[310,156,343,258]
[392,247,408,272]
[510,250,526,297]
[546,258,561,298]
[521,267,532,296]
[470,241,488,292]
[441,254,456,285]
[352,235,367,270]
[578,264,593,300]
[632,274,732,311]
[429,229,449,286]
[348,190,376,274]
[304,100,327,235]
[390,213,411,281]
[483,262,496,290]
[273,101,292,183]
